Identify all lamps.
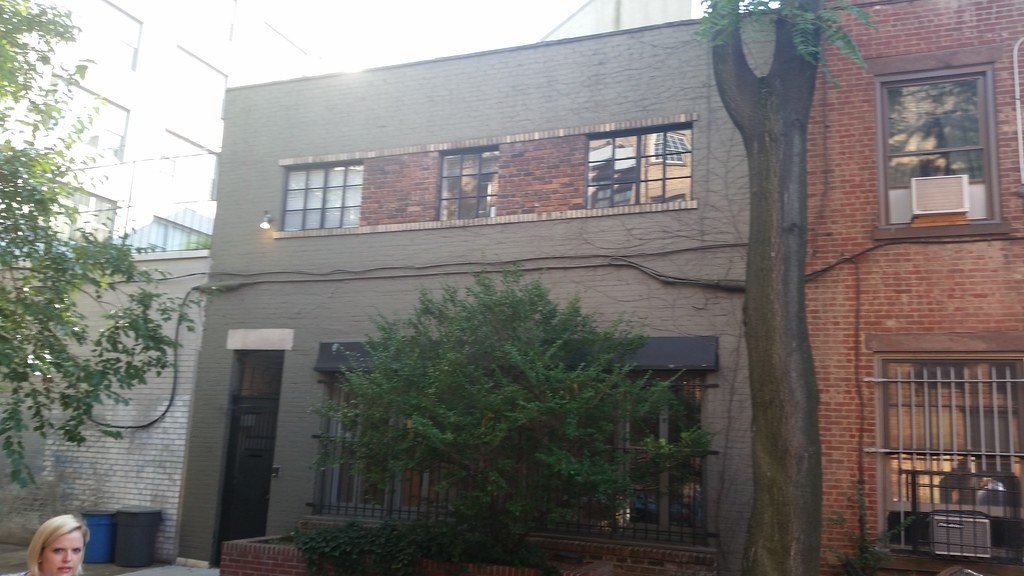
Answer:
[260,213,274,229]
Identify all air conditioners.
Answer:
[926,514,992,559]
[910,174,970,215]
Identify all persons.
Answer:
[0,512,91,576]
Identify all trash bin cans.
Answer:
[82,510,114,564]
[114,509,164,566]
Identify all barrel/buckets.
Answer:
[113,504,162,567]
[80,509,116,563]
